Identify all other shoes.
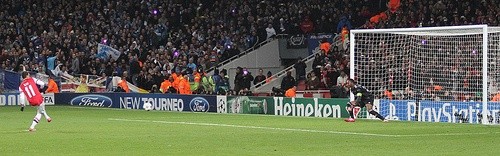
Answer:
[29,128,36,132]
[345,118,354,122]
[383,119,388,123]
[48,118,51,122]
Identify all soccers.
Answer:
[144,103,151,112]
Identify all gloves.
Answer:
[43,89,45,94]
[21,107,24,111]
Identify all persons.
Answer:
[344,79,388,123]
[18,72,52,132]
[0,0,500,101]
